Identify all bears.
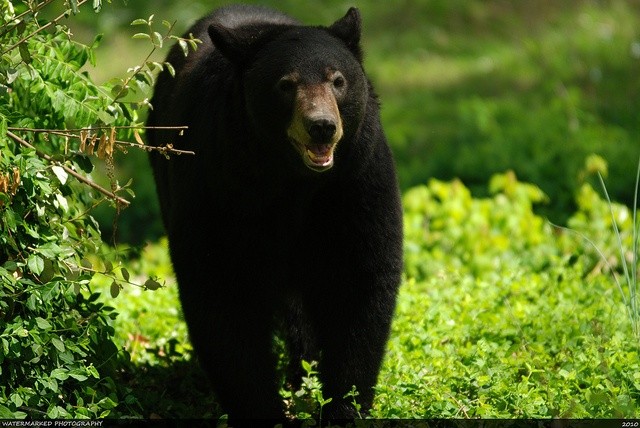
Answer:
[143,1,404,427]
